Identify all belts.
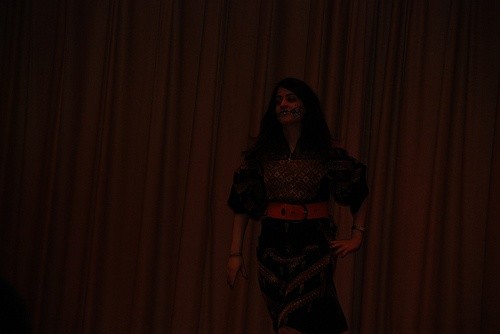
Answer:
[264,202,330,221]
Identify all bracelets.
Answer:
[229,253,242,257]
[351,225,365,232]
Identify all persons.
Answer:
[226,79,370,334]
